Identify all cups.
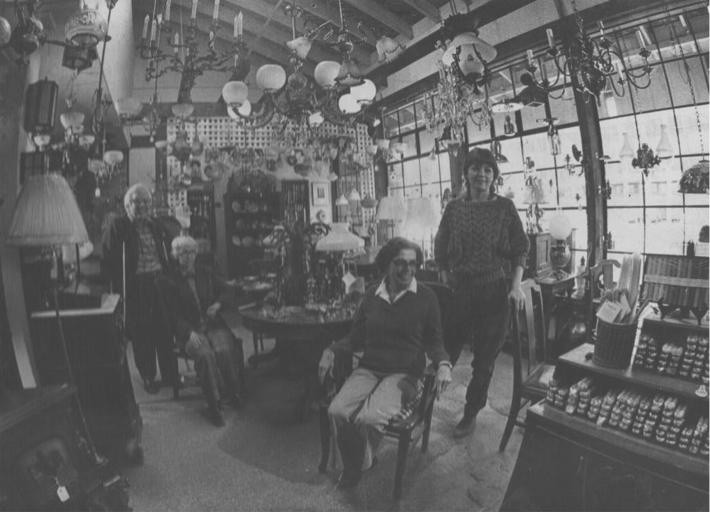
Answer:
[592,316,637,370]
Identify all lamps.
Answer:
[663,2,710,196]
[286,2,406,65]
[1,0,139,205]
[406,199,441,261]
[548,207,573,280]
[2,174,103,310]
[141,120,414,206]
[486,99,672,207]
[318,223,367,309]
[443,1,497,85]
[422,65,486,158]
[374,195,407,241]
[225,1,377,127]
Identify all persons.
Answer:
[167,235,247,426]
[102,182,179,393]
[434,149,530,440]
[313,238,454,503]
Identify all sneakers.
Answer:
[454,416,476,437]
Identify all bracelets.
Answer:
[439,360,451,368]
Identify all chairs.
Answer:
[316,282,455,500]
[498,281,562,454]
[556,258,620,344]
[154,278,243,408]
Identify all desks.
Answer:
[1,382,132,511]
[240,304,362,379]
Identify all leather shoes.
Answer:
[144,377,158,394]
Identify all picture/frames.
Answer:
[311,181,330,207]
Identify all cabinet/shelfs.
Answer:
[31,293,144,464]
[223,177,309,266]
[497,253,709,512]
[186,184,217,259]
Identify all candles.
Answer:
[547,29,556,50]
[191,1,197,19]
[209,31,215,49]
[213,1,219,19]
[235,55,238,68]
[151,21,157,41]
[615,63,624,82]
[239,12,244,34]
[527,49,535,67]
[234,16,238,38]
[174,33,180,52]
[636,30,648,51]
[142,15,148,40]
[165,0,171,20]
